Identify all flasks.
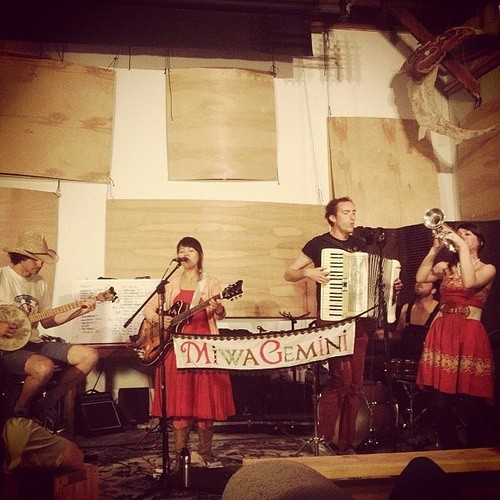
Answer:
[179,447,191,488]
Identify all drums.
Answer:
[316,377,399,450]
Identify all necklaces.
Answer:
[457,259,480,269]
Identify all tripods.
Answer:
[351,243,438,452]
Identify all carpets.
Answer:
[85,436,312,500]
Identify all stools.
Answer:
[0,365,75,441]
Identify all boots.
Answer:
[197,427,219,464]
[169,425,191,477]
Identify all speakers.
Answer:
[75,391,124,436]
[119,388,150,422]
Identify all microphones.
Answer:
[354,226,383,236]
[173,257,190,262]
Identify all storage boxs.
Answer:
[35,462,99,500]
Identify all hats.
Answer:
[2,232,60,263]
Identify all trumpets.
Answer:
[424,207,464,249]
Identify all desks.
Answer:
[81,344,138,394]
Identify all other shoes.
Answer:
[339,448,357,454]
[34,401,57,432]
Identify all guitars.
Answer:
[131,280,244,368]
[0,286,117,351]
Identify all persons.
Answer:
[144,237,235,463]
[285,197,403,455]
[0,232,100,417]
[0,395,84,500]
[416,222,496,451]
[375,282,440,341]
